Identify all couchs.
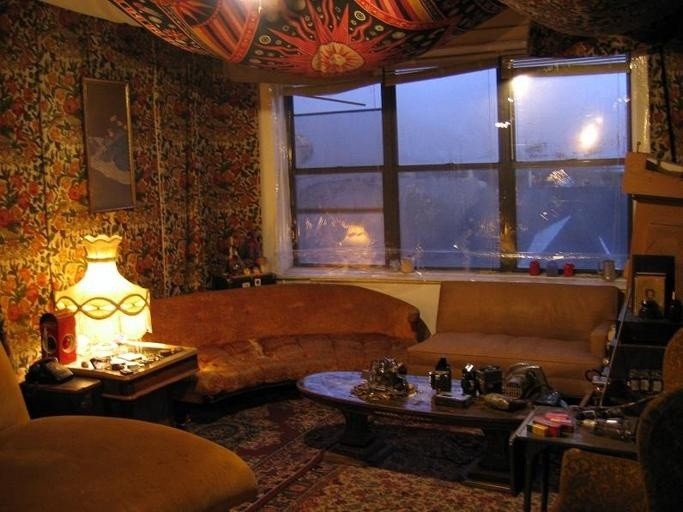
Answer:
[151,284,421,423]
[406,281,619,401]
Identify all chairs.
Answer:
[549,328,683,511]
[0,343,257,512]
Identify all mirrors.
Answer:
[82,78,139,214]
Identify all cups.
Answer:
[529,261,575,276]
[597,260,618,281]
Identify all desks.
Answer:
[511,406,639,512]
[70,342,200,431]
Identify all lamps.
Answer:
[54,233,153,344]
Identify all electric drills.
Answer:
[479,392,527,411]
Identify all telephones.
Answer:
[25,357,74,384]
